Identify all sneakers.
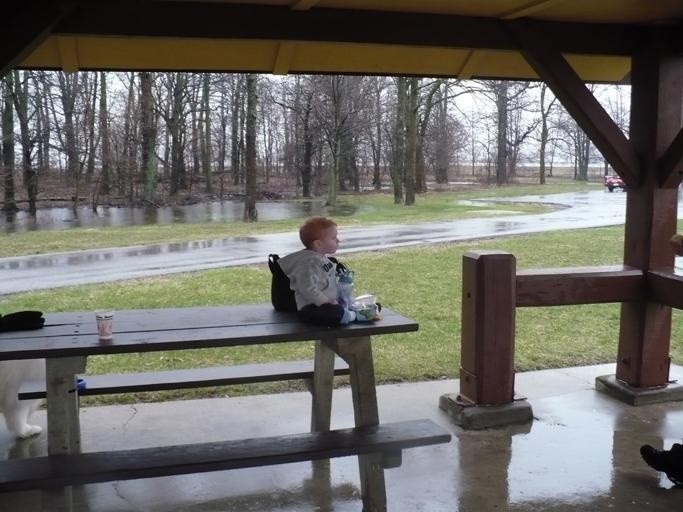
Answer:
[639,443,683,486]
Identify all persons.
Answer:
[277,217,381,327]
[639,231,683,487]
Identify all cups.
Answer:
[95,309,115,340]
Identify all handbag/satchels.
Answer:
[268,254,344,313]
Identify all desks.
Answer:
[0,300,418,510]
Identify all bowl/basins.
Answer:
[352,304,377,321]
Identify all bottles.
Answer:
[337,270,354,307]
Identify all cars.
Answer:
[604,175,628,192]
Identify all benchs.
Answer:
[1,418,452,511]
[16,358,350,401]
